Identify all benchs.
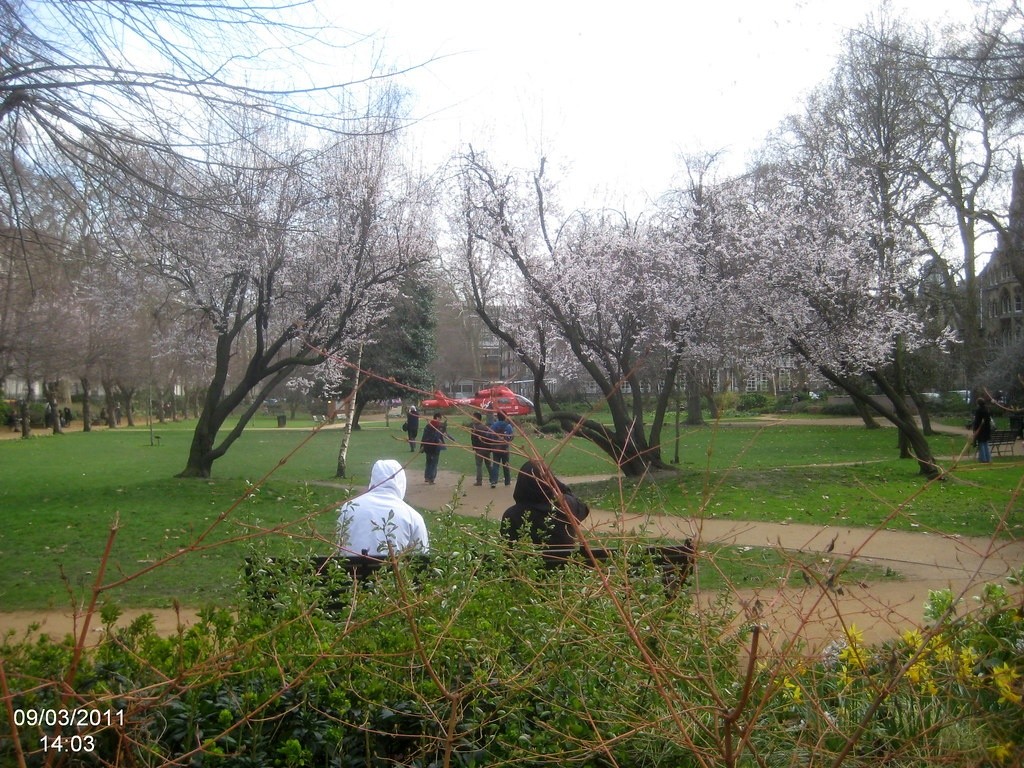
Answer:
[336,413,347,423]
[313,415,320,425]
[322,415,326,421]
[971,428,1019,459]
[244,540,696,613]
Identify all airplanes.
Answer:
[420,384,534,417]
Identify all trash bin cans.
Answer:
[1009,414,1024,436]
[277,415,286,428]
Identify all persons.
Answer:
[471,412,496,488]
[100,408,109,425]
[59,407,72,427]
[114,406,123,425]
[486,410,513,485]
[420,413,456,485]
[44,399,57,428]
[336,460,429,557]
[8,410,21,432]
[973,398,991,463]
[500,460,589,551]
[407,406,419,451]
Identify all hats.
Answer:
[409,405,417,411]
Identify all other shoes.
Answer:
[505,481,512,486]
[490,482,496,489]
[429,481,436,484]
[473,482,482,486]
[414,444,416,448]
[425,478,429,482]
[411,448,414,452]
